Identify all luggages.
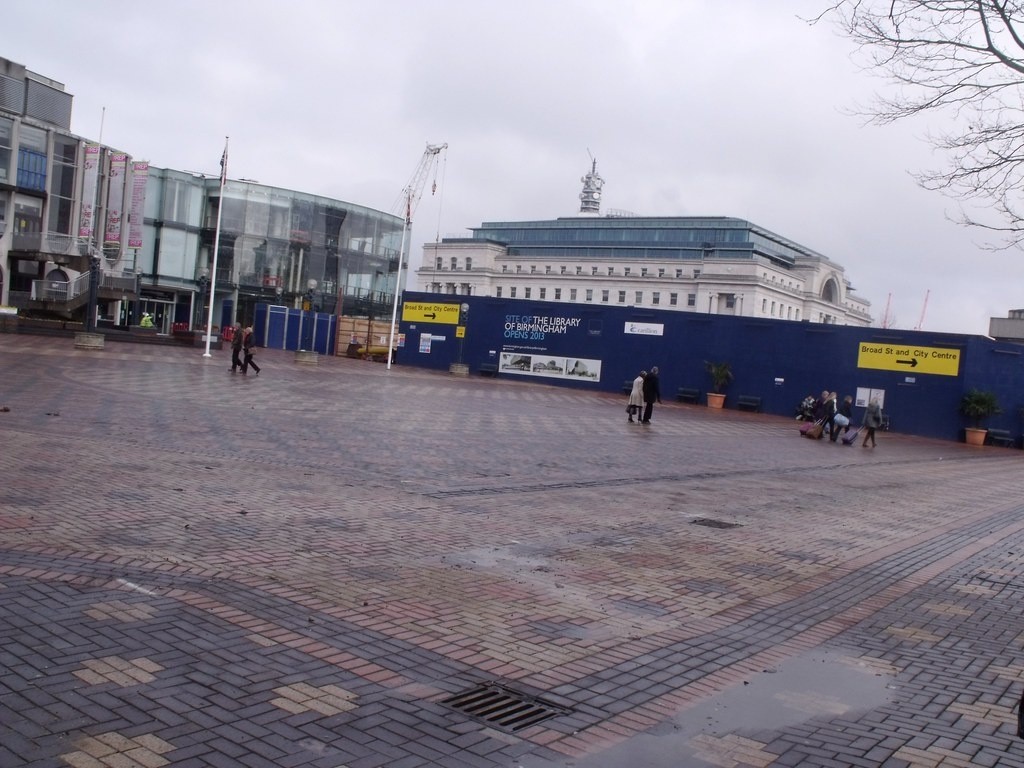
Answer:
[841,425,866,444]
[805,415,828,439]
[800,421,814,435]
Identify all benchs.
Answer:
[736,395,761,413]
[878,414,890,431]
[478,363,498,378]
[676,387,700,405]
[622,380,633,396]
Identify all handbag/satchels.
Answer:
[626,406,637,415]
[834,413,849,426]
[247,347,257,355]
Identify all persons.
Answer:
[240,327,261,375]
[228,322,244,372]
[628,371,647,422]
[643,366,663,424]
[795,390,882,448]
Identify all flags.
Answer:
[220,147,227,185]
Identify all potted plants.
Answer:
[959,387,1005,446]
[703,360,734,409]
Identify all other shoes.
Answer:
[228,368,236,371]
[638,419,642,423]
[642,419,650,424]
[240,370,247,373]
[240,364,244,370]
[628,418,634,422]
[256,368,260,374]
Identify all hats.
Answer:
[651,367,658,374]
